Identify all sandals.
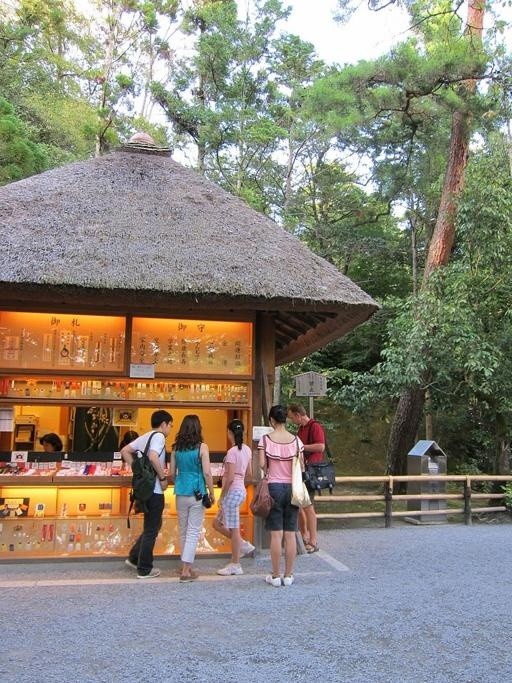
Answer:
[304,541,319,553]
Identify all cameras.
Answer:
[217,480,222,487]
[202,494,211,508]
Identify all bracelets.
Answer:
[159,476,166,481]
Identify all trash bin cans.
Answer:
[407,440,447,521]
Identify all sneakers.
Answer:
[125,559,160,579]
[217,563,242,576]
[266,575,294,587]
[180,569,199,583]
[241,541,255,557]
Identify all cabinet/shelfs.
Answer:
[0,451,252,563]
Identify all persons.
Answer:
[286,403,326,554]
[119,431,139,454]
[170,414,216,583]
[256,404,305,586]
[121,409,173,578]
[39,433,63,453]
[212,419,256,575]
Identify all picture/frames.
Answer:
[110,403,139,428]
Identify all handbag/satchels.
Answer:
[303,462,335,490]
[250,479,274,516]
[291,457,311,508]
[131,457,156,503]
